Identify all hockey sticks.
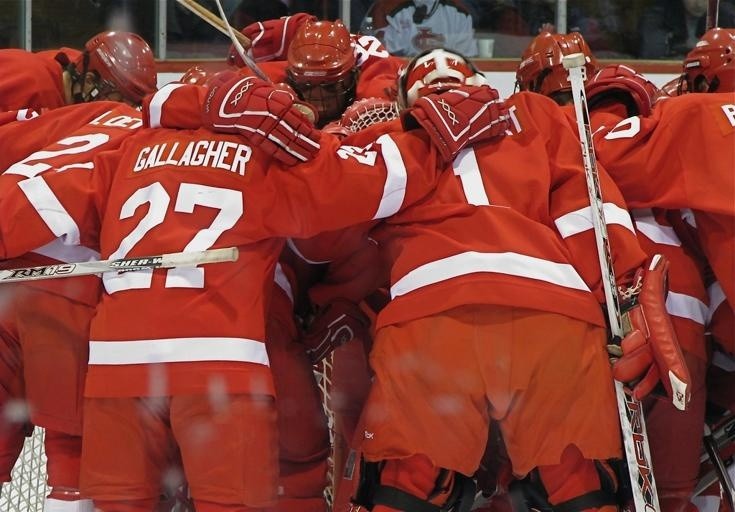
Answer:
[218,1,279,88]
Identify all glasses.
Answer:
[290,81,345,94]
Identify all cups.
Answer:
[475,39,494,57]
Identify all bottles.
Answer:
[358,16,375,36]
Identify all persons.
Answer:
[1,0,735,511]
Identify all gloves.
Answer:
[610,252,692,412]
[201,73,322,166]
[583,62,658,119]
[400,84,510,169]
[293,297,372,365]
[228,13,318,68]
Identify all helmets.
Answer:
[286,21,357,87]
[399,47,485,108]
[73,30,157,106]
[682,27,735,93]
[516,31,599,96]
